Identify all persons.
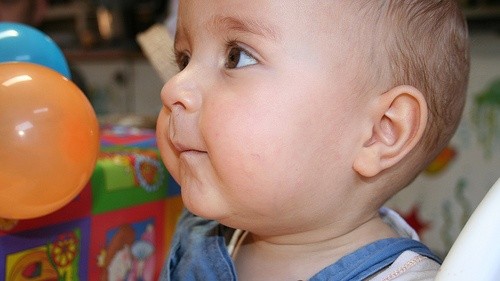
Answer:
[154,0,471,281]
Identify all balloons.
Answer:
[0,62,97,223]
[0,22,70,79]
